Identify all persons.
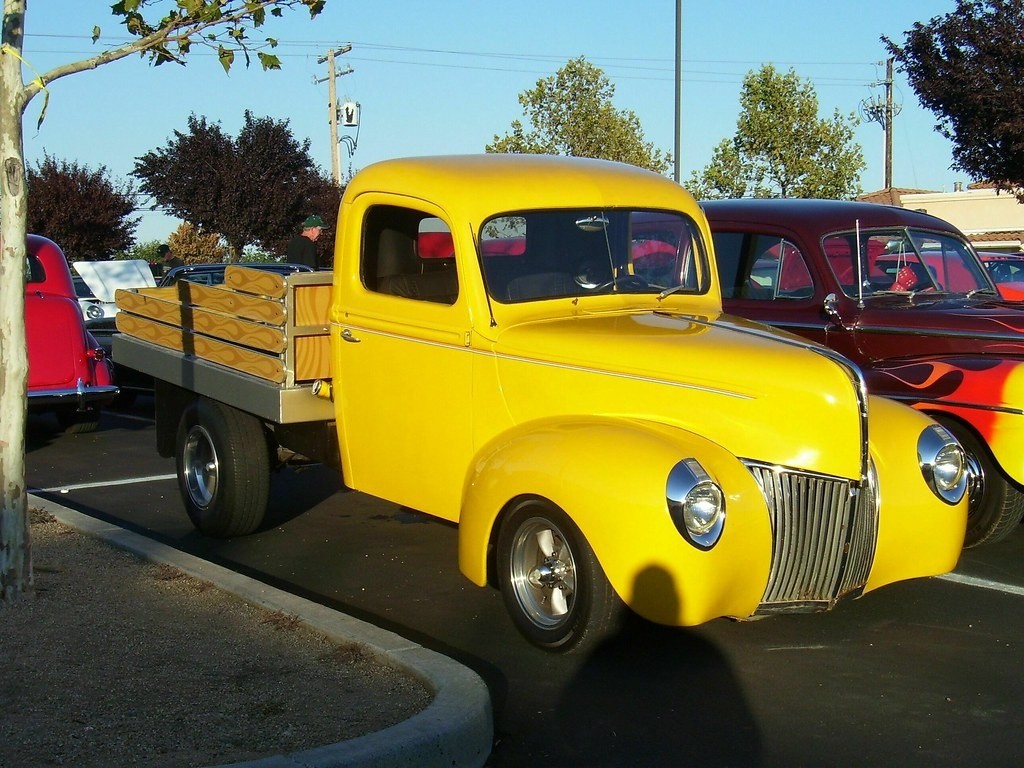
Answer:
[155,244,185,287]
[284,215,331,272]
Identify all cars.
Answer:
[624,197,1022,555]
[872,246,1024,312]
[24,232,122,445]
[68,261,178,334]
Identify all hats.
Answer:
[156,245,169,253]
[301,215,330,229]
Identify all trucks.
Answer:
[106,154,968,657]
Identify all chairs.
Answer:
[504,272,589,298]
[381,269,495,304]
